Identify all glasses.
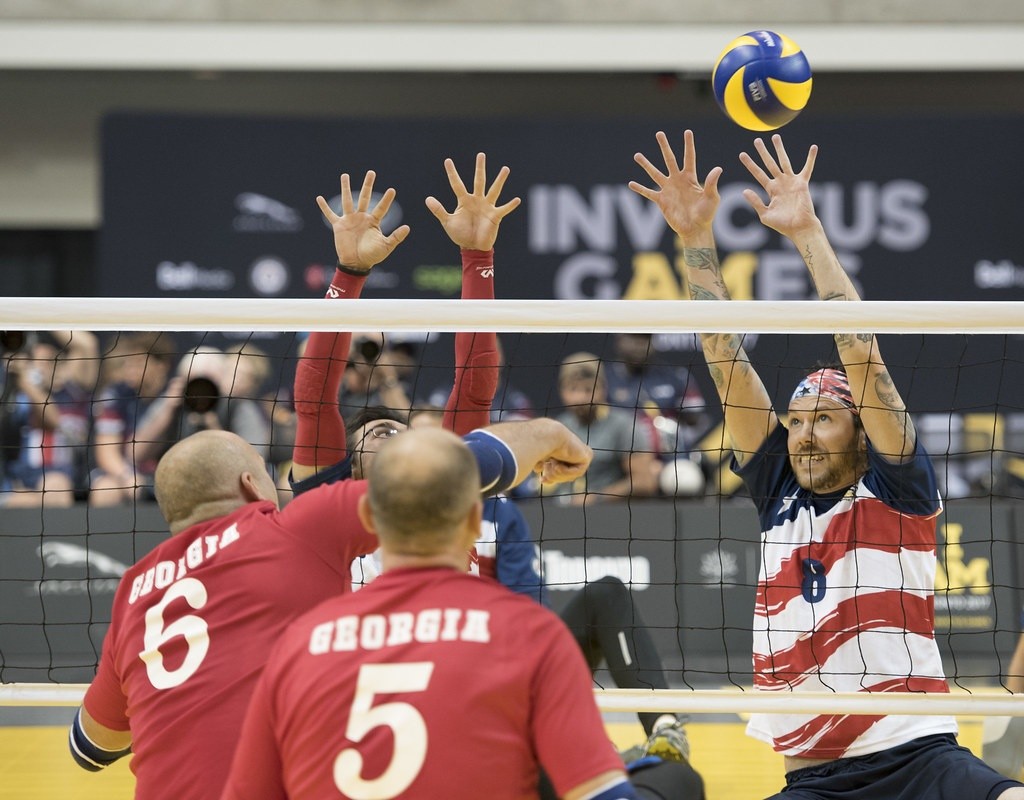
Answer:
[349,426,398,458]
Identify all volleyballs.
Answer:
[711,29,815,135]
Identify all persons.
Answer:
[982,627,1023,781]
[3,331,711,507]
[69,412,589,800]
[625,129,1024,800]
[281,151,525,500]
[215,425,787,800]
[470,493,691,762]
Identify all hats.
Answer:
[559,351,606,383]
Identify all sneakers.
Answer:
[641,715,690,765]
